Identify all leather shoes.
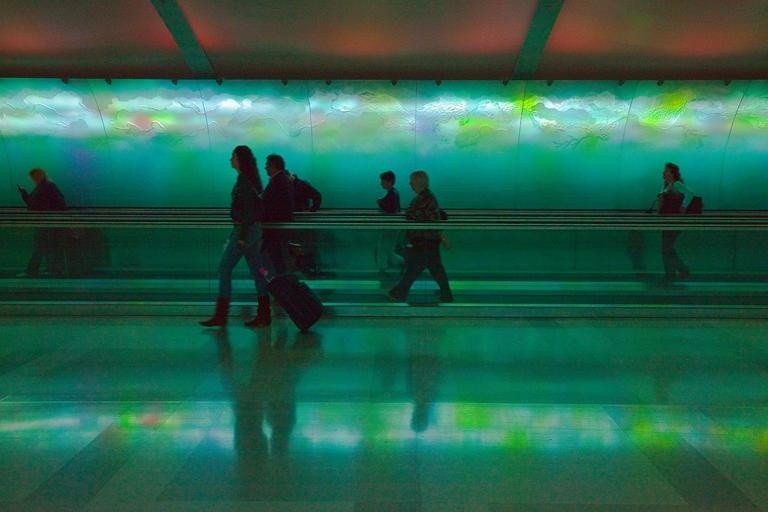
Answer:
[15,271,35,277]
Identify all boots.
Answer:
[244,295,271,327]
[198,298,229,326]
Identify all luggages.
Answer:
[236,242,324,334]
[286,239,320,279]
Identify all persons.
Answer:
[376,171,400,270]
[200,145,322,329]
[19,167,67,278]
[657,162,694,283]
[385,170,455,304]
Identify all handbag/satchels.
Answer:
[437,222,457,249]
